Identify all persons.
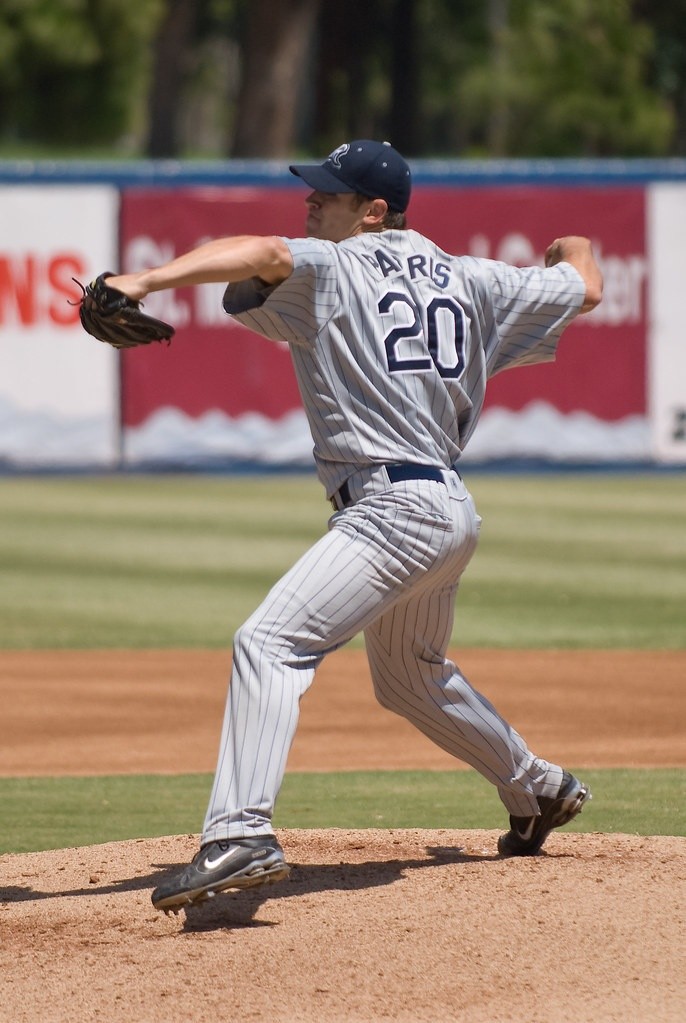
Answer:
[71,138,603,917]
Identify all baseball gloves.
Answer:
[79,271,175,349]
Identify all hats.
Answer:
[289,141,412,213]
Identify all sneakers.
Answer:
[498,769,593,857]
[151,834,293,916]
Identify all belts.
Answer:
[330,465,462,511]
